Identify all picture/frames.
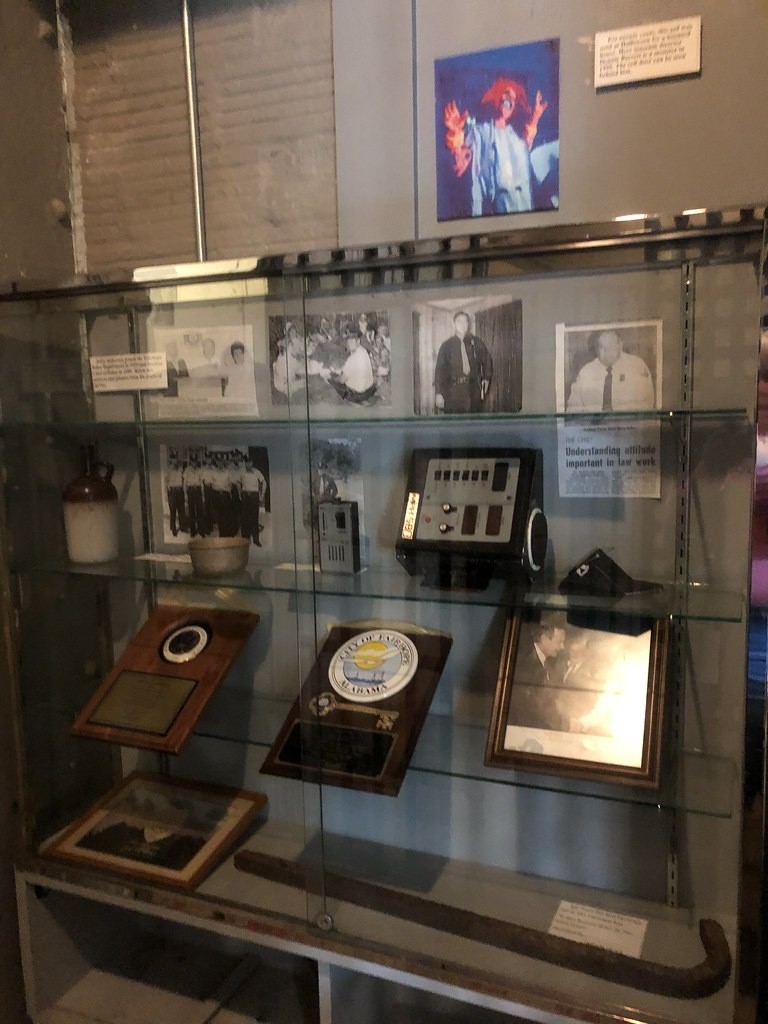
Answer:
[483,599,669,789]
[41,768,269,891]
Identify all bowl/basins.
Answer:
[190,536,253,577]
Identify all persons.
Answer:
[164,450,266,547]
[508,619,648,740]
[444,79,549,217]
[312,461,338,542]
[161,338,253,399]
[273,313,391,408]
[435,312,493,415]
[566,330,654,411]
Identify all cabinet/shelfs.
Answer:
[0,214,768,1024]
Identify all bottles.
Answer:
[60,447,121,565]
